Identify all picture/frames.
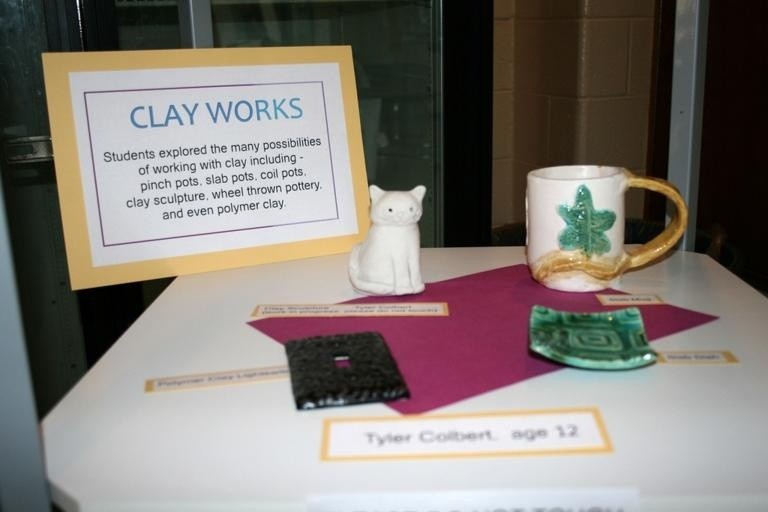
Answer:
[40,46,375,290]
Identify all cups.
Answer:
[523,162,690,294]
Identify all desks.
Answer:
[40,244,765,508]
